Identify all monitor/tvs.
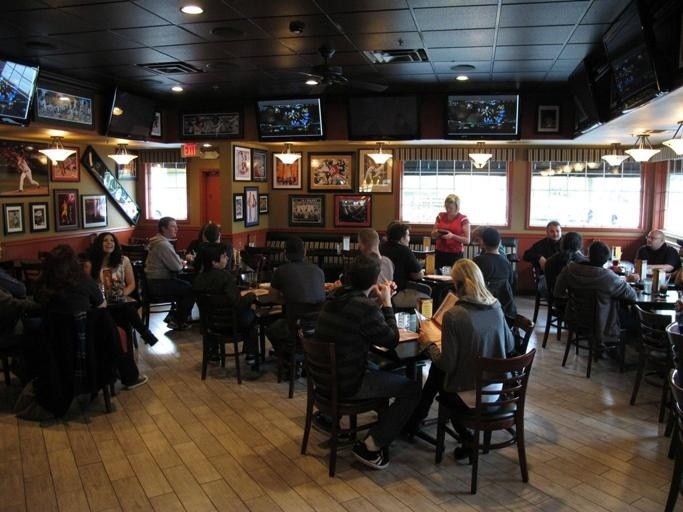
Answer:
[344,93,422,141]
[603,2,668,113]
[104,85,158,142]
[444,92,522,140]
[254,98,326,142]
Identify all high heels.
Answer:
[454,433,474,464]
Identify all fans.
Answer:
[267,47,389,93]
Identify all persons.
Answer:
[266,237,325,377]
[185,225,260,366]
[145,218,196,331]
[523,221,579,300]
[310,221,518,469]
[553,240,637,340]
[544,232,586,307]
[430,195,470,268]
[1,231,160,422]
[634,230,681,273]
[15,154,39,193]
[60,199,68,224]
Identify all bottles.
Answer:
[608,242,672,299]
[178,247,195,267]
[230,247,241,266]
[395,312,420,335]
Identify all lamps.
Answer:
[600,143,630,166]
[662,121,683,156]
[623,134,661,162]
[468,142,492,164]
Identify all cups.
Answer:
[441,265,451,277]
[247,272,259,291]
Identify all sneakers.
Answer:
[127,375,148,389]
[311,411,352,442]
[351,440,389,469]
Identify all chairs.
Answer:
[299,335,390,479]
[443,310,534,456]
[0,243,142,426]
[528,244,682,510]
[133,243,300,398]
[435,347,537,496]
[392,268,455,311]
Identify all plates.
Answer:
[324,283,334,292]
[260,282,271,289]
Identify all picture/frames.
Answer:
[0,137,141,235]
[178,108,245,139]
[149,111,162,138]
[535,103,562,135]
[233,144,393,227]
[32,83,95,130]
